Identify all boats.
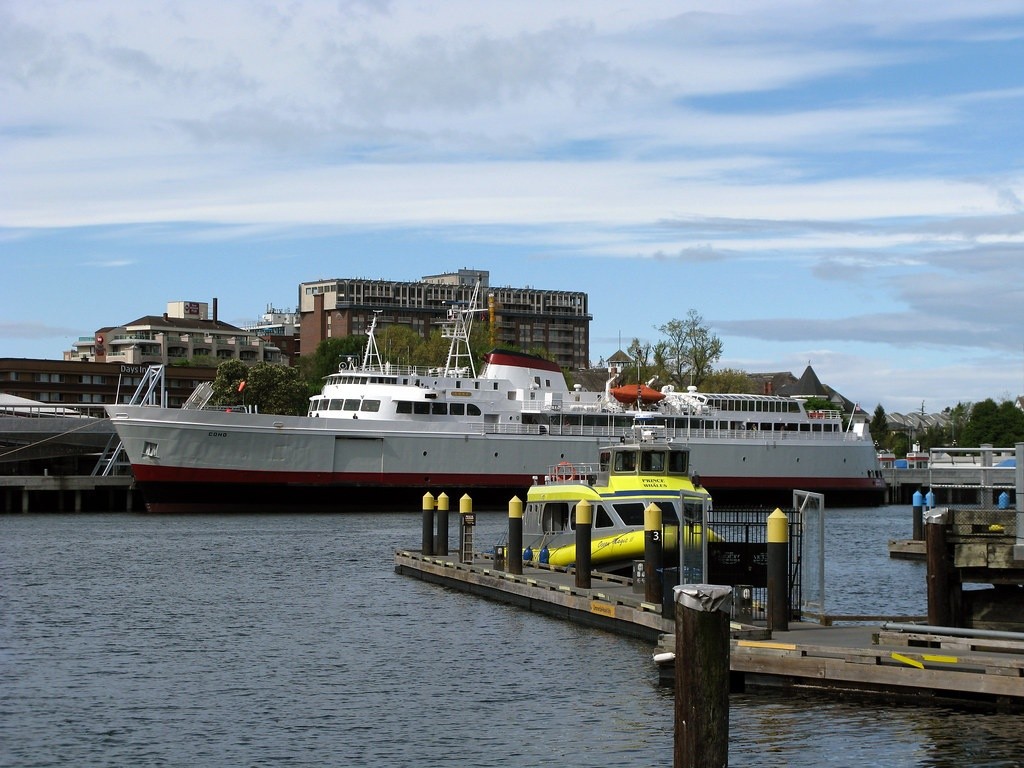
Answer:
[496,408,727,581]
[609,382,666,404]
[102,281,895,510]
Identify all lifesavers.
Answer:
[554,462,576,482]
